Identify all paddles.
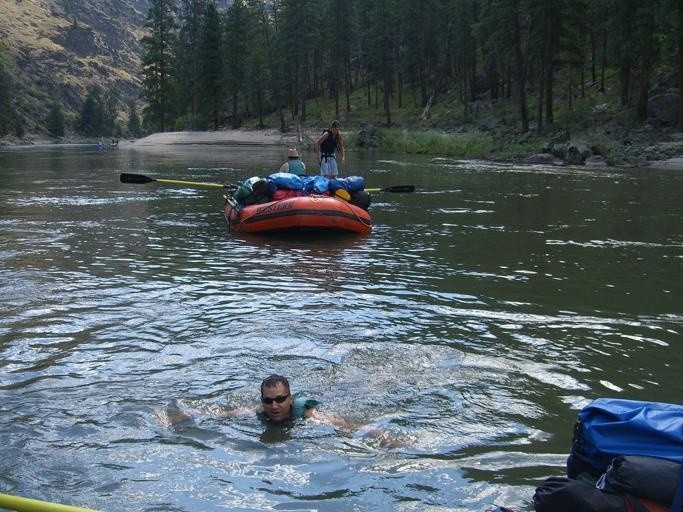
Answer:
[120,173,230,189]
[363,185,415,193]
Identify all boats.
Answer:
[224,174,373,234]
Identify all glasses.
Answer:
[261,394,290,405]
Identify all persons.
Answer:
[159,373,392,447]
[279,149,306,177]
[315,120,345,178]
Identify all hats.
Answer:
[287,149,301,156]
